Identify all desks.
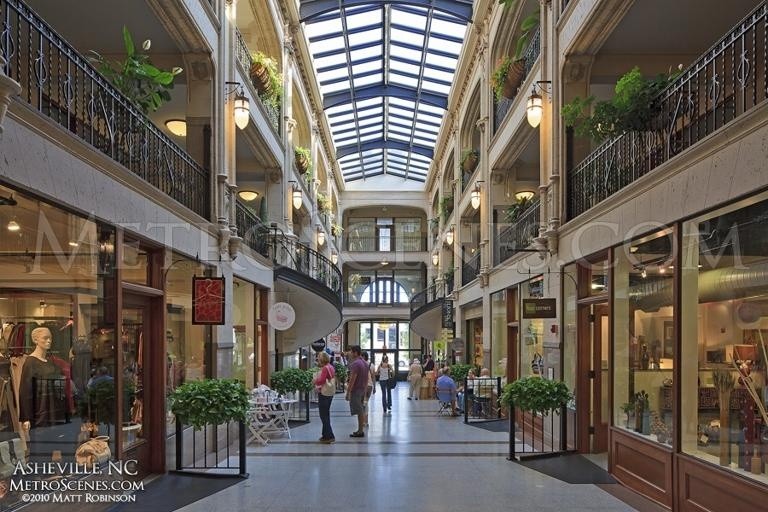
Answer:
[661,387,761,427]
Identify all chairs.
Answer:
[433,385,493,418]
[246,397,297,447]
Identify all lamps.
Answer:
[315,224,324,246]
[239,190,259,201]
[432,80,551,266]
[225,81,249,130]
[165,119,186,137]
[288,180,302,209]
[331,248,338,264]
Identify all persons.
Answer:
[436,366,465,415]
[406,357,423,400]
[436,368,444,399]
[87,365,114,392]
[343,344,370,438]
[311,350,336,445]
[88,367,102,388]
[361,350,377,428]
[377,355,395,412]
[471,367,495,417]
[18,325,72,474]
[422,355,428,371]
[455,368,476,413]
[421,354,434,377]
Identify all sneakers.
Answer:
[349,430,364,437]
[319,433,335,442]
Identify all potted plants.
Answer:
[251,54,281,105]
[293,146,312,175]
[495,56,527,99]
[317,193,332,213]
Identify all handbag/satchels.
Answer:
[321,365,336,396]
[74,435,111,469]
[386,364,396,389]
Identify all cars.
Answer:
[375,354,408,367]
